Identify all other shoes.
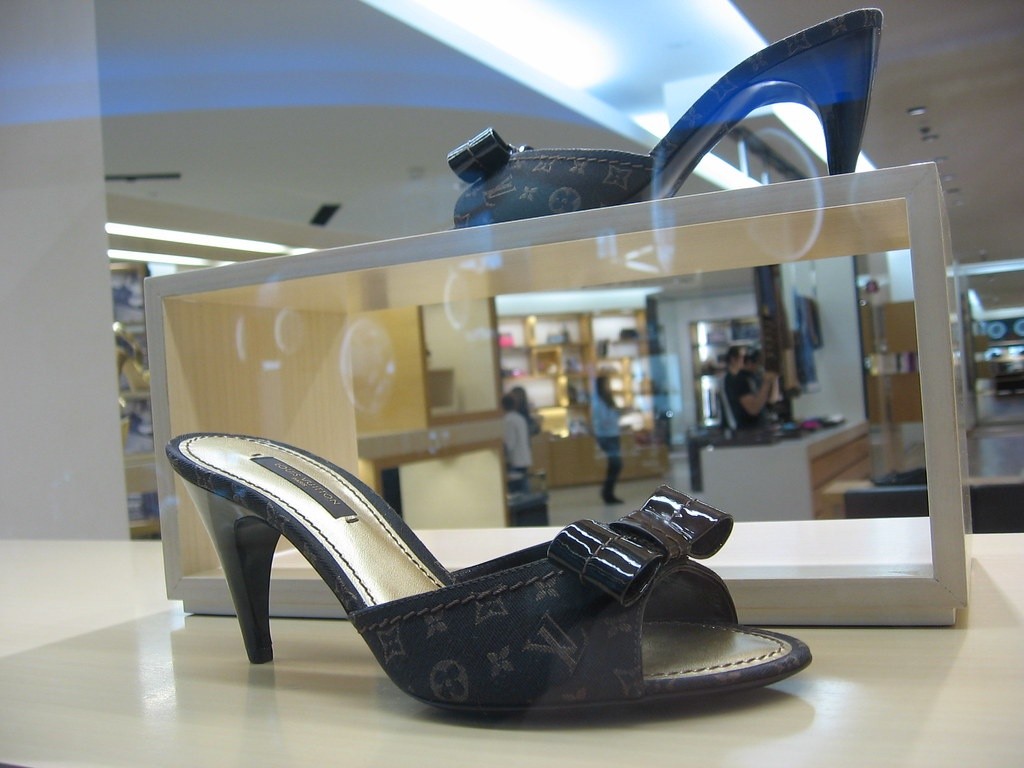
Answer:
[600,491,625,505]
[870,468,927,487]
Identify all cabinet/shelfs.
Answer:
[497,310,659,419]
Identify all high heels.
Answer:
[166,433,812,717]
[447,8,884,229]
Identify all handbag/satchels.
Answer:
[499,332,513,348]
[621,330,638,339]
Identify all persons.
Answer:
[590,376,622,504]
[501,387,539,493]
[719,347,780,430]
